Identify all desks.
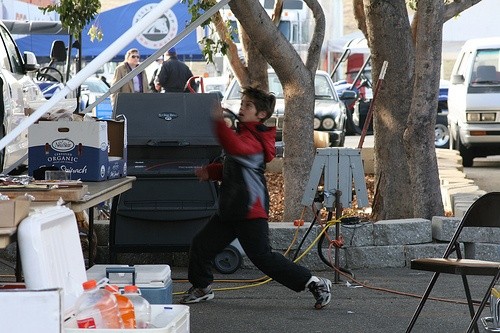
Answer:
[0,171,137,282]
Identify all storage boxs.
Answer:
[62,298,191,333]
[27,116,128,180]
[86,264,174,305]
[0,194,31,229]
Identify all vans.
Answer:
[447,37,500,168]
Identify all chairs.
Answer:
[476,66,497,84]
[405,192,500,333]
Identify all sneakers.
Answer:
[307,276,331,309]
[180,285,215,304]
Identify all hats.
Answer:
[168,47,176,54]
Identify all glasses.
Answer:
[130,55,139,58]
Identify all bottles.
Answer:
[75,277,151,329]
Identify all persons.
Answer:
[179,85,333,310]
[100,48,195,111]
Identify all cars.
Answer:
[31,70,228,121]
[215,70,358,150]
[1,24,68,177]
[330,75,452,148]
